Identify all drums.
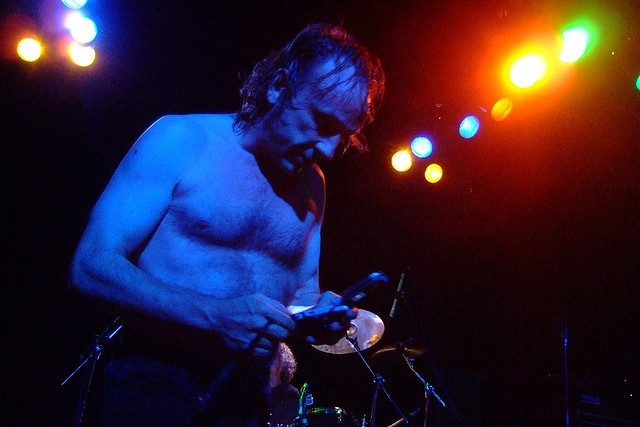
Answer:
[303,307,387,403]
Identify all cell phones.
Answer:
[293,272,393,318]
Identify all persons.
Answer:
[261,340,300,427]
[67,23,386,426]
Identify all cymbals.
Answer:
[370,338,437,370]
